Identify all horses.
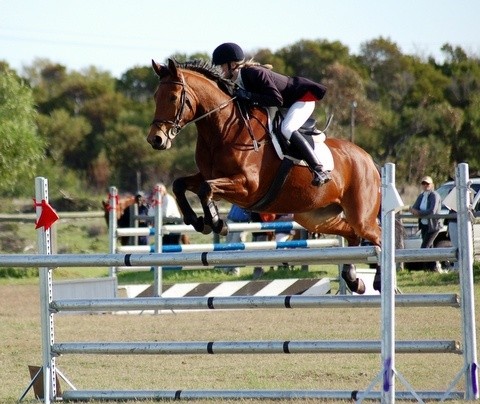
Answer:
[147,58,403,295]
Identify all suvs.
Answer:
[361,177,480,275]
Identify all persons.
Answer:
[149,184,182,244]
[212,42,332,186]
[409,176,441,248]
[118,183,320,271]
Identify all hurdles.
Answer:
[0,163,480,404]
